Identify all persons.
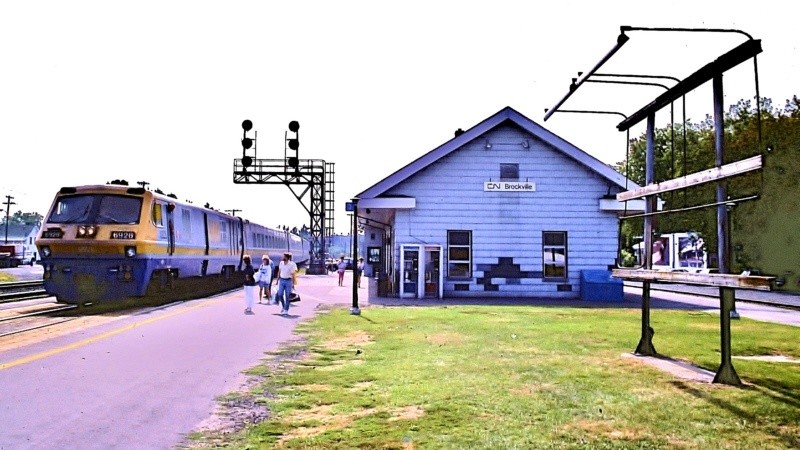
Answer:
[275,252,298,315]
[336,256,346,286]
[651,237,667,265]
[357,257,364,288]
[242,256,256,312]
[256,254,274,305]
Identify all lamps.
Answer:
[485,138,493,150]
[521,140,530,150]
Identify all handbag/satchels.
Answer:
[253,268,260,283]
[288,287,300,302]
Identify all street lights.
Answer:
[0,208,9,245]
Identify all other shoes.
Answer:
[268,298,271,304]
[284,309,288,317]
[280,306,285,313]
[245,307,251,312]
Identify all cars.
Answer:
[324,259,354,272]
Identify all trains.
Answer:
[632,232,710,274]
[33,183,310,306]
